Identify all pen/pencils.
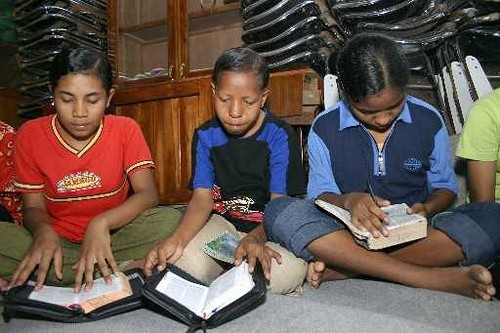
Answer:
[369,185,381,210]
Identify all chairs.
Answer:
[239,0,500,133]
[13,1,106,119]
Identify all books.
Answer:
[315,198,428,250]
[2,268,149,322]
[142,258,267,333]
[203,231,241,264]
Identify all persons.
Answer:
[454,87,498,203]
[0,47,183,292]
[143,47,308,297]
[264,33,499,302]
[0,121,24,227]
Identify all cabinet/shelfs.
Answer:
[107,0,322,206]
